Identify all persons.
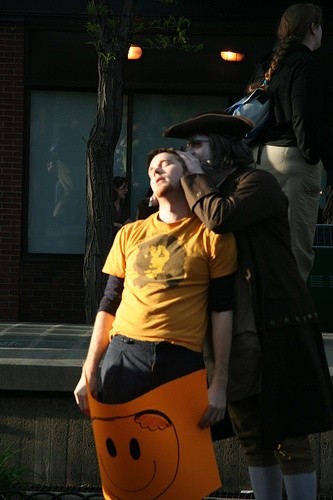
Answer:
[247,2,325,284]
[136,113,333,500]
[111,175,133,244]
[72,147,245,500]
[136,190,159,223]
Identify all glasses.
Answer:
[183,138,210,148]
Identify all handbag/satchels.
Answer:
[222,88,270,145]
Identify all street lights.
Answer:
[120,43,144,243]
[215,43,251,116]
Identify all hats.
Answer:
[162,111,255,138]
